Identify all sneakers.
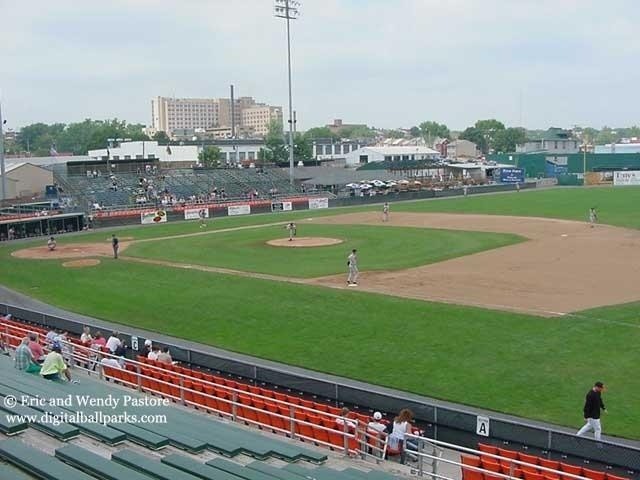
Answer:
[71,379,81,386]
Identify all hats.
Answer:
[373,411,382,421]
[52,343,61,349]
[595,382,603,388]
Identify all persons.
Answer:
[587,208,597,228]
[1,313,177,384]
[335,404,421,457]
[110,232,121,260]
[283,221,297,242]
[81,164,308,210]
[196,209,211,228]
[574,382,610,453]
[378,202,391,223]
[346,249,359,286]
[5,209,75,253]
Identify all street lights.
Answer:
[274,0,301,187]
[579,144,593,186]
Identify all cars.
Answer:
[618,138,631,144]
[344,178,421,191]
